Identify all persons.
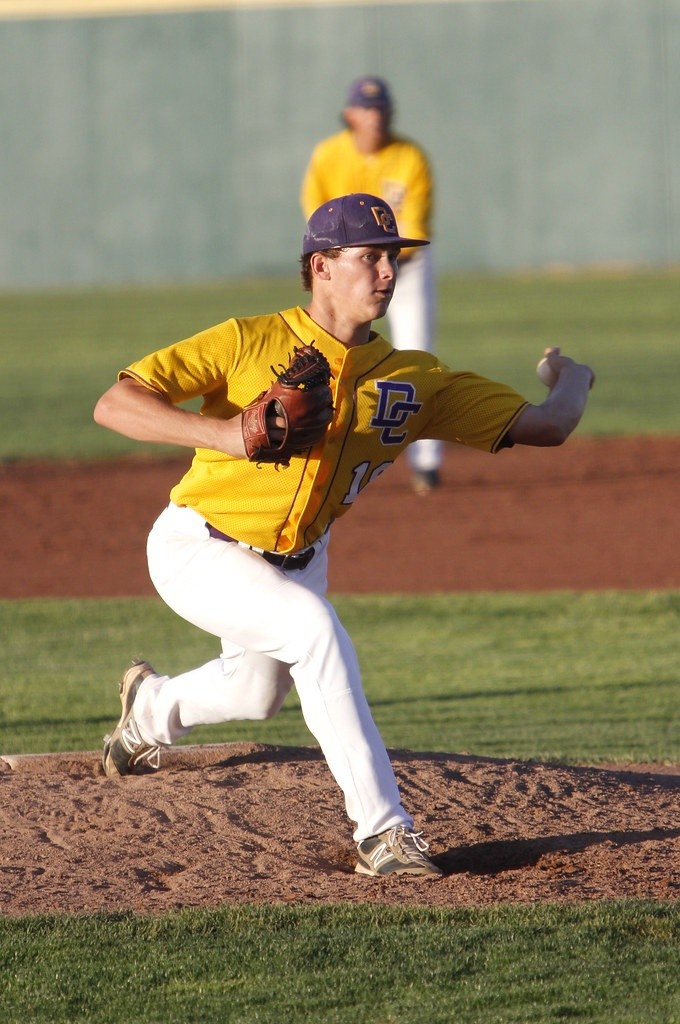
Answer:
[92,194,595,881]
[300,73,441,491]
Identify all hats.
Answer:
[302,194,431,254]
[345,77,390,109]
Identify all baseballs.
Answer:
[536,358,551,387]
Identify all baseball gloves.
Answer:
[242,345,335,462]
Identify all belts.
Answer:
[204,521,316,571]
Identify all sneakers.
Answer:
[102,658,161,778]
[354,826,443,880]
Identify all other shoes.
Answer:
[412,467,436,495]
